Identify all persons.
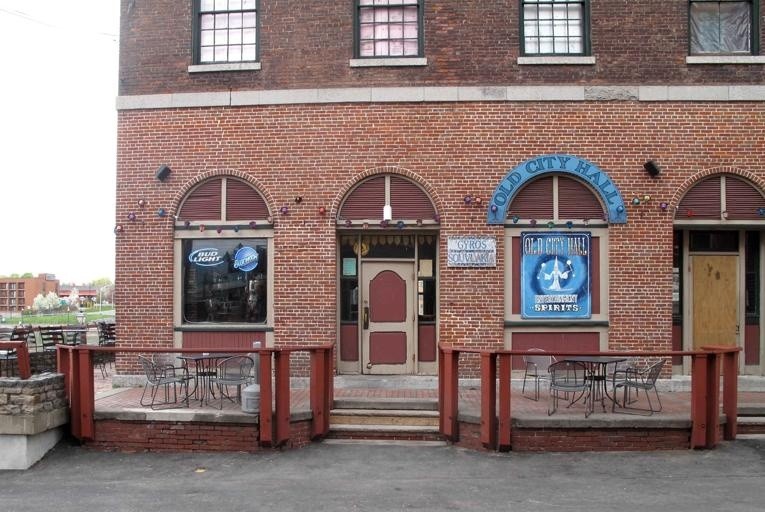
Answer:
[17,321,23,328]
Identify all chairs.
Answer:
[520,347,667,419]
[138,350,255,410]
[0,318,116,380]
